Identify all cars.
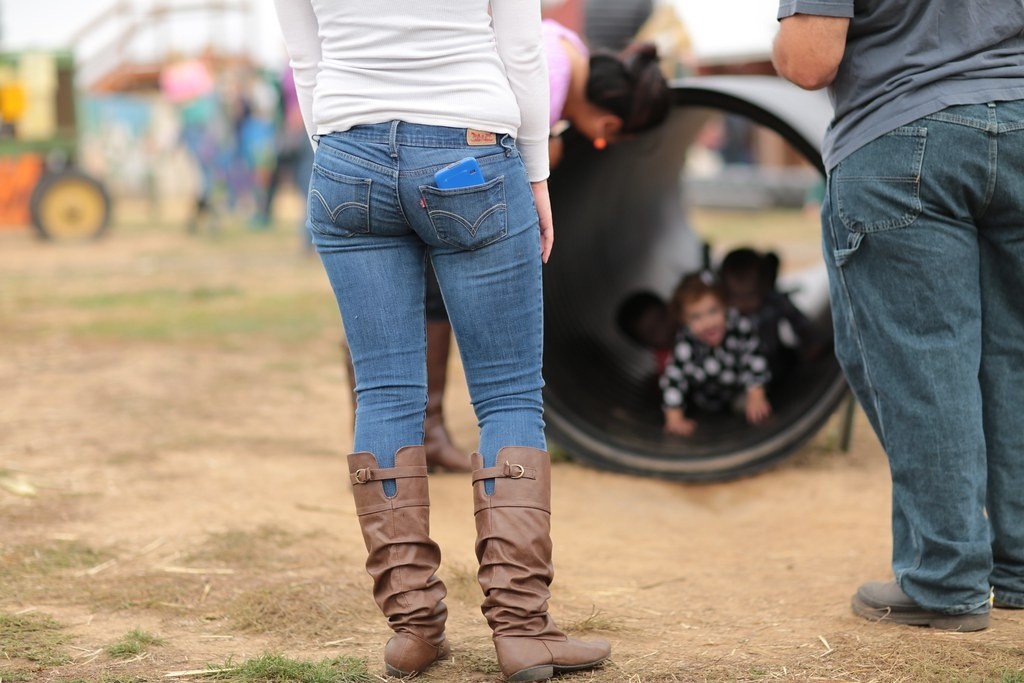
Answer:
[0,130,107,241]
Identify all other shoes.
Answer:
[851,580,989,631]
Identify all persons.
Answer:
[343,20,669,473]
[276,0,612,683]
[772,0,1024,631]
[614,248,808,432]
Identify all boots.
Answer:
[424,320,477,471]
[341,337,358,432]
[346,446,451,676]
[472,444,610,683]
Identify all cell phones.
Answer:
[433,157,485,187]
